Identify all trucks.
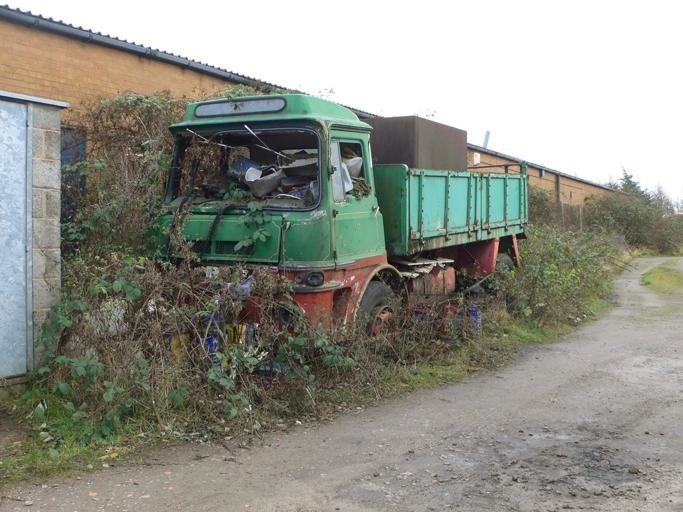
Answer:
[149,92,531,347]
